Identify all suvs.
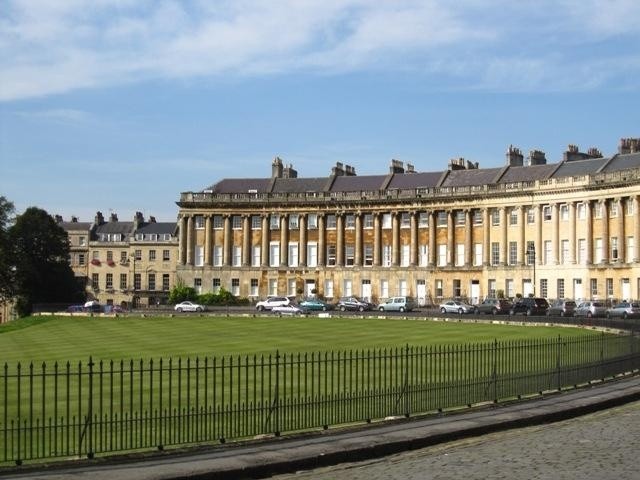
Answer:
[474,297,514,315]
[509,296,550,316]
[255,296,294,312]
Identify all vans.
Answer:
[545,299,577,317]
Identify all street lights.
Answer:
[524,242,536,297]
[128,253,138,308]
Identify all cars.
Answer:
[173,300,208,313]
[377,295,414,313]
[271,302,309,315]
[605,302,640,319]
[298,298,332,312]
[336,296,370,312]
[572,300,608,318]
[66,300,127,313]
[438,300,476,314]
